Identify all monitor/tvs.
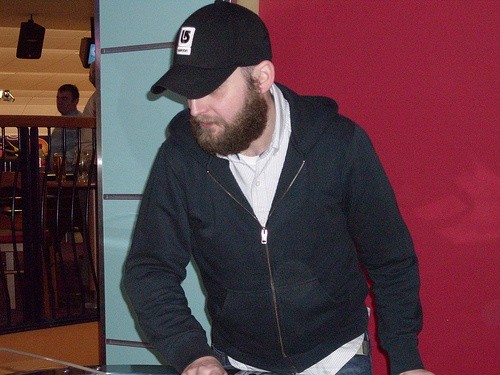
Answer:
[78,37,95,68]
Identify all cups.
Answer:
[54,153,66,183]
[75,150,88,182]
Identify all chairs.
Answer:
[0,150,100,323]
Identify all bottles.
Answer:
[39,144,46,174]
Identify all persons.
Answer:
[79,62,96,181]
[47,85,82,248]
[120,4,434,375]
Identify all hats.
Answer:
[151,3,273,99]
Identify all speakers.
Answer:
[16,22,45,59]
